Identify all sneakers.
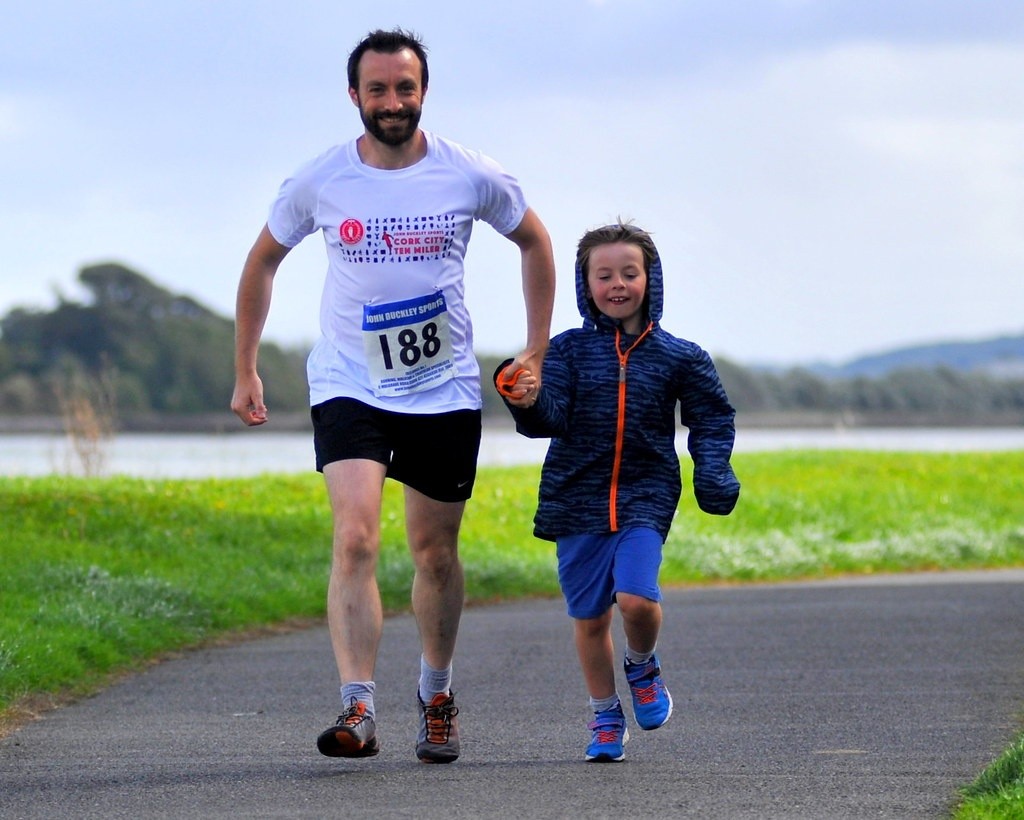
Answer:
[583,656,673,765]
[316,687,459,766]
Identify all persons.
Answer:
[493,221,746,766]
[227,27,555,766]
